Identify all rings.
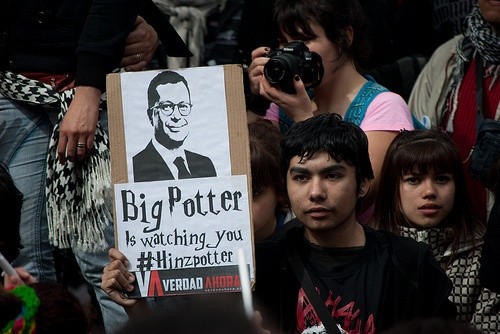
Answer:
[137,53,141,62]
[77,143,85,147]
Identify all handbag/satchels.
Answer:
[469,119,497,177]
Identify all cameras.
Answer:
[262,40,324,94]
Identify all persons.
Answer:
[0,0,500,334]
